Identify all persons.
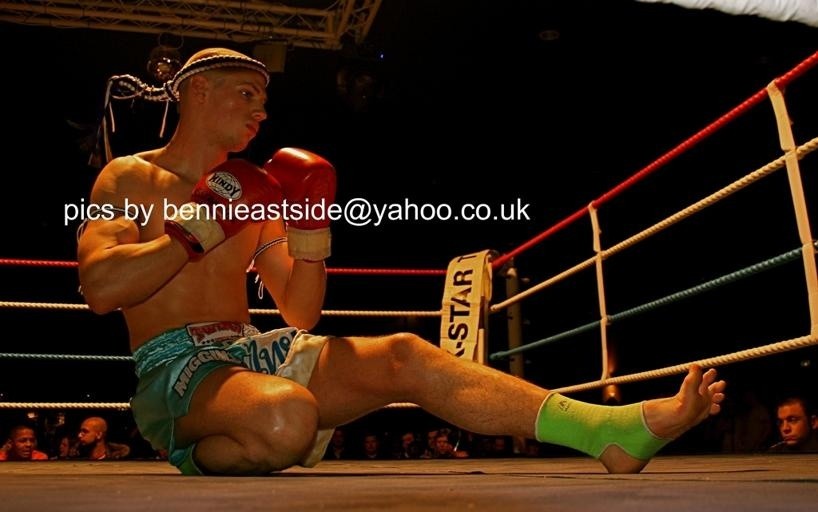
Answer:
[77,47,726,476]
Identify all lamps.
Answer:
[243,21,291,77]
[145,31,184,83]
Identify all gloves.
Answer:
[164,157,283,264]
[263,148,337,264]
